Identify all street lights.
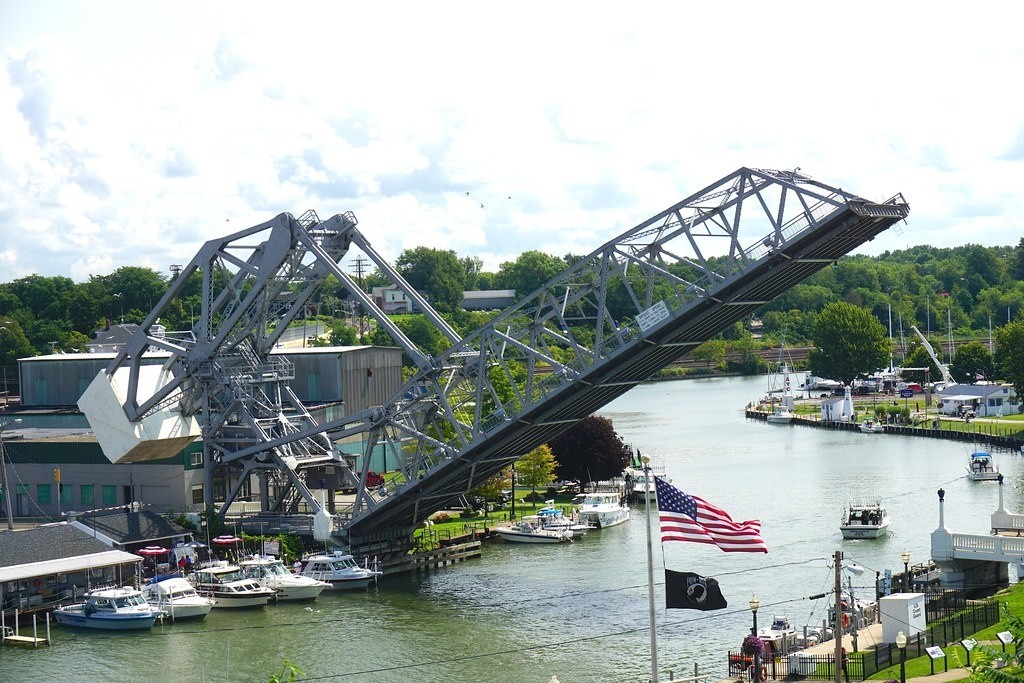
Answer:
[510,461,517,519]
[748,595,764,683]
[901,549,911,592]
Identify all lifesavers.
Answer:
[842,612,850,627]
[759,666,767,681]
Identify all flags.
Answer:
[665,569,728,611]
[654,476,768,554]
[976,373,984,380]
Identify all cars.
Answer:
[484,488,512,505]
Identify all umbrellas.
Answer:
[134,545,171,574]
[210,534,243,544]
[183,541,208,553]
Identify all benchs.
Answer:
[204,501,272,513]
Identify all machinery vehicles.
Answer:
[958,405,975,419]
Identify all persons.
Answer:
[178,555,191,570]
[625,473,632,485]
[875,412,883,425]
[841,647,852,683]
[981,465,985,473]
[293,558,302,575]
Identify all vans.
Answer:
[341,471,385,493]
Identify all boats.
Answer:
[963,449,1003,482]
[121,573,218,621]
[183,565,279,609]
[765,405,794,424]
[632,471,674,500]
[290,548,385,592]
[839,500,891,540]
[856,418,885,434]
[234,554,336,603]
[494,491,631,544]
[50,587,169,630]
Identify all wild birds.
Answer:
[226,219,229,222]
[466,191,511,208]
